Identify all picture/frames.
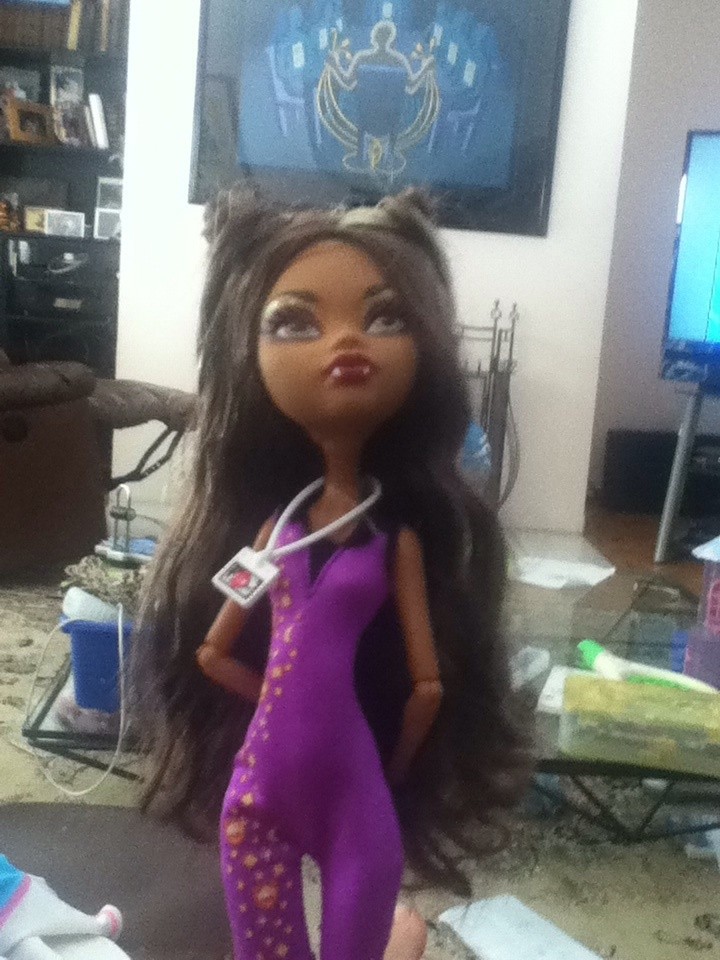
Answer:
[188,0,573,238]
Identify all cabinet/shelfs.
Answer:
[0,45,125,350]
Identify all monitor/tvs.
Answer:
[189,0,569,237]
[658,132,720,389]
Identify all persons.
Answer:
[128,182,532,960]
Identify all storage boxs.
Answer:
[59,617,137,711]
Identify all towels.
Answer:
[1,851,136,956]
